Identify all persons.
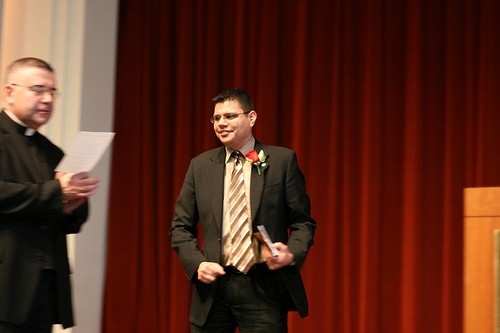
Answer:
[170,88,317,333]
[0,57,101,333]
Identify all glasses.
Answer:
[10,83,60,98]
[210,110,251,122]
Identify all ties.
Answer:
[230,149,257,274]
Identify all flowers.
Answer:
[245,149,267,176]
